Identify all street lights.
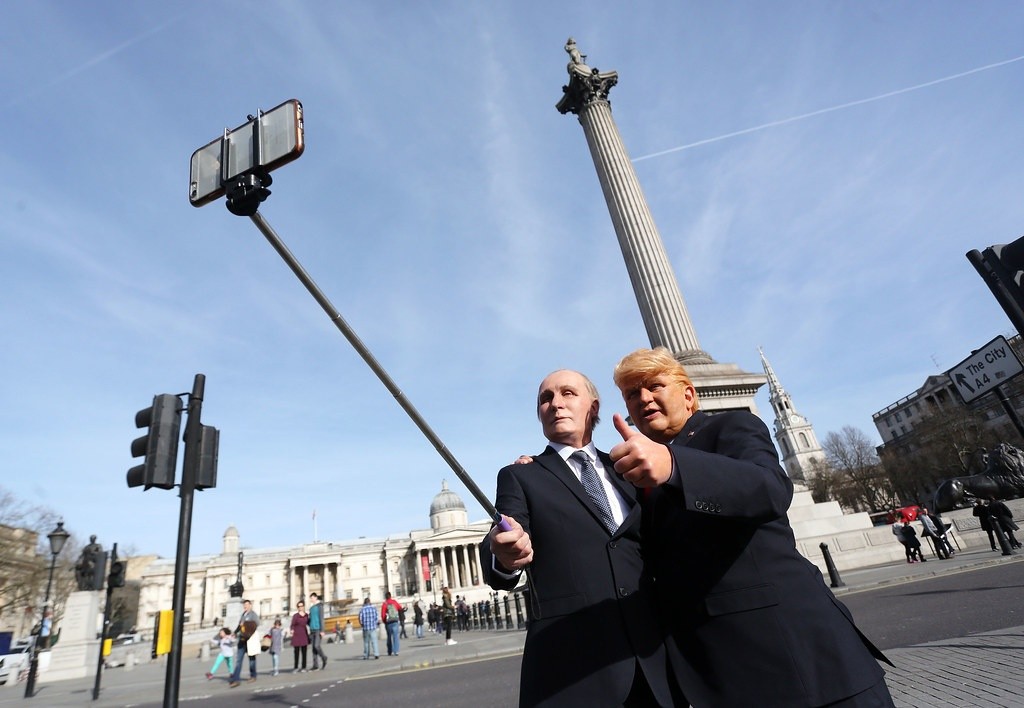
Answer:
[23,521,71,697]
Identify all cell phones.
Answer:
[188,99,305,208]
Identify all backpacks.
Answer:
[384,600,398,622]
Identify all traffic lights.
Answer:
[126,393,183,491]
[110,560,127,587]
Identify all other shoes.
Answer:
[1013,543,1022,549]
[309,667,319,672]
[446,638,457,645]
[247,676,256,683]
[375,656,379,659]
[205,672,213,679]
[292,668,306,674]
[322,657,330,669]
[907,559,914,563]
[229,680,241,687]
[388,652,399,656]
[992,548,999,552]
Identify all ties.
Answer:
[570,450,618,535]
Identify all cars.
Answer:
[0,646,31,684]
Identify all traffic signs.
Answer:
[948,335,1024,404]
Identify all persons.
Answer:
[479,369,692,708]
[565,38,582,65]
[891,495,1021,564]
[205,588,466,687]
[514,346,899,708]
[22,610,52,665]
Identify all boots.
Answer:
[911,552,927,562]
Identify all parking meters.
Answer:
[151,610,184,708]
[92,638,112,700]
